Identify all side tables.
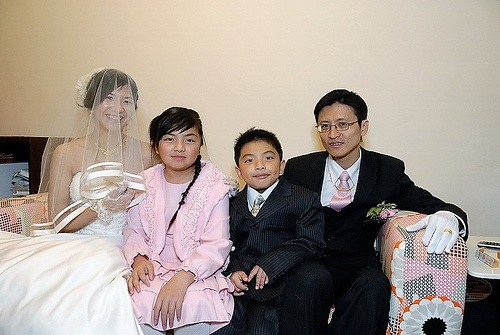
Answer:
[468,236,500,279]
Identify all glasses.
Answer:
[315,121,359,132]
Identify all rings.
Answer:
[444,228,452,236]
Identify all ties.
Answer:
[329,171,352,212]
[251,195,265,217]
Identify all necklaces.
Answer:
[327,160,355,191]
[90,133,127,159]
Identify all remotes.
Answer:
[477,241,500,249]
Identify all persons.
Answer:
[120,107,235,333]
[0,68,240,334]
[279,89,469,335]
[209,126,326,335]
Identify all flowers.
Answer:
[366,201,398,223]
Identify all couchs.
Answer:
[0,193,468,335]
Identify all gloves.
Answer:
[406,211,459,254]
